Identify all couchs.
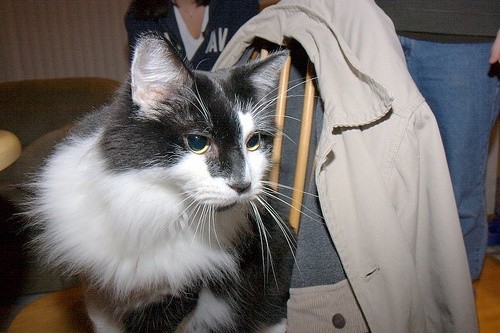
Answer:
[0,78,126,333]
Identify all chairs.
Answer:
[7,0,478,333]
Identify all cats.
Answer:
[0,21,320,333]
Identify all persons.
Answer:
[124,0,260,71]
[376,0,500,280]
